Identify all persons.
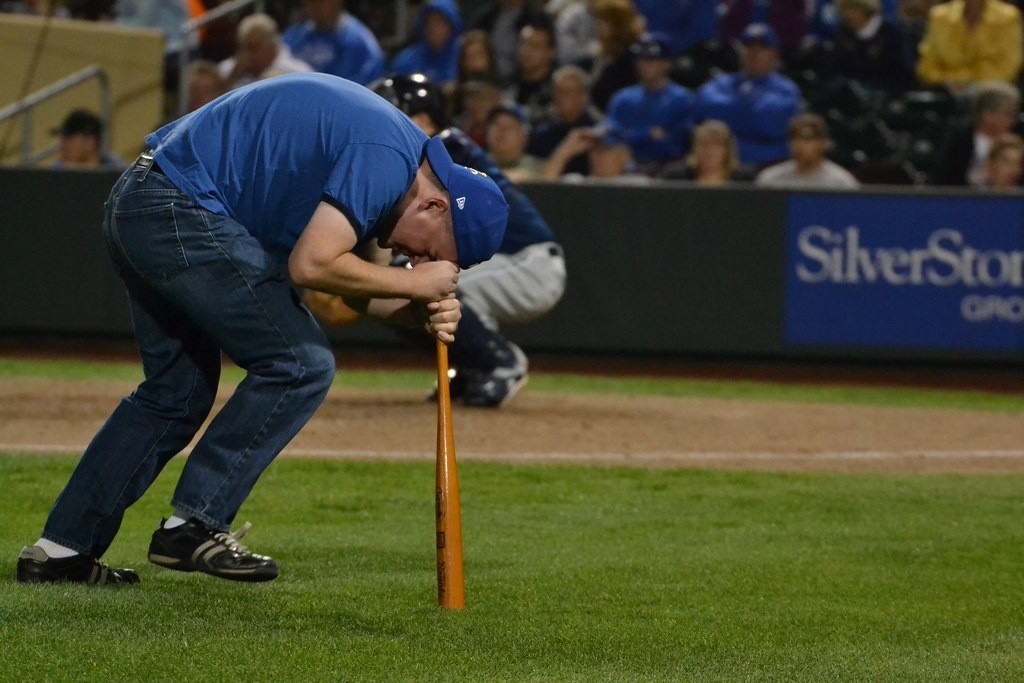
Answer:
[444,27,503,152]
[796,0,919,122]
[752,114,860,189]
[716,0,817,76]
[914,1,1024,99]
[279,0,386,91]
[481,104,596,184]
[692,19,814,178]
[14,71,511,588]
[156,8,316,131]
[388,0,463,84]
[934,76,1024,188]
[503,14,566,104]
[362,67,568,413]
[547,1,718,112]
[599,28,702,177]
[47,108,128,170]
[663,119,756,187]
[466,0,552,83]
[524,64,619,179]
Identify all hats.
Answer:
[50,110,103,137]
[426,134,511,270]
[635,34,671,58]
[788,114,825,140]
[743,24,775,46]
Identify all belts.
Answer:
[136,155,165,175]
[547,247,559,255]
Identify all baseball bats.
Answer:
[434,334,466,611]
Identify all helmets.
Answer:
[374,72,447,124]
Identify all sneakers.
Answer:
[468,342,528,407]
[432,367,476,402]
[16,545,140,587]
[147,516,279,582]
